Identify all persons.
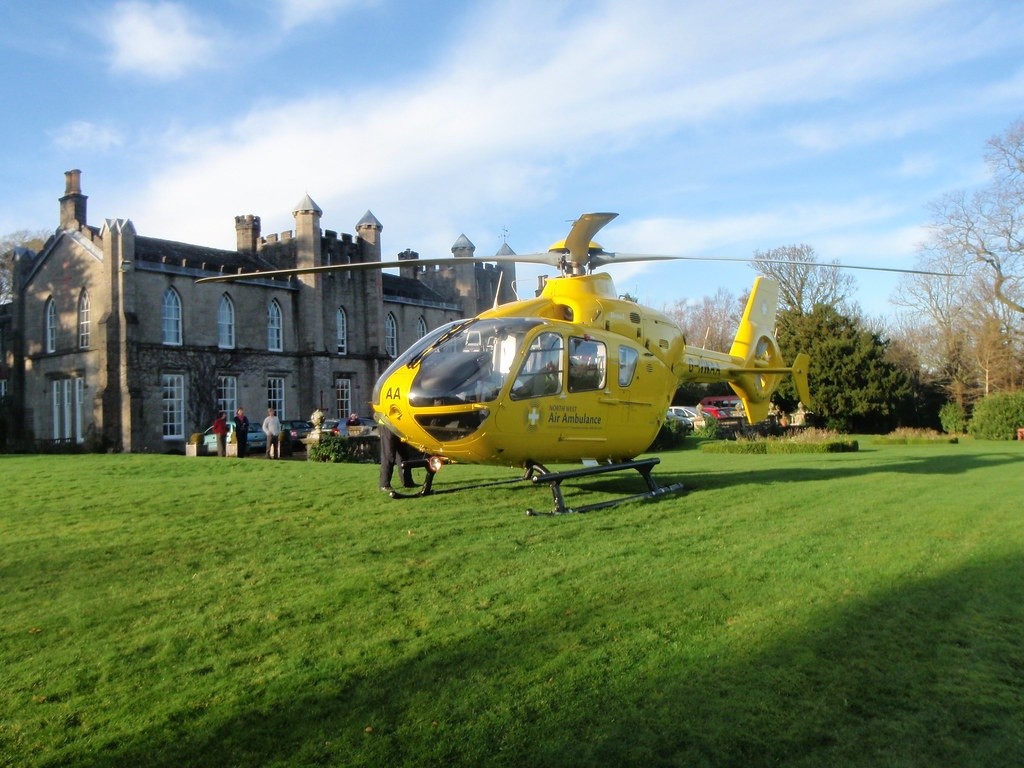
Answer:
[234,408,249,458]
[378,419,421,491]
[346,414,360,427]
[262,408,281,459]
[212,411,228,457]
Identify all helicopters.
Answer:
[194,211,969,520]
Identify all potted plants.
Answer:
[270,429,292,459]
[185,433,209,457]
[226,432,238,456]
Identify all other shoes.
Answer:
[380,487,395,498]
[404,483,417,487]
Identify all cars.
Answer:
[666,394,742,430]
[280,416,379,457]
[203,421,267,456]
